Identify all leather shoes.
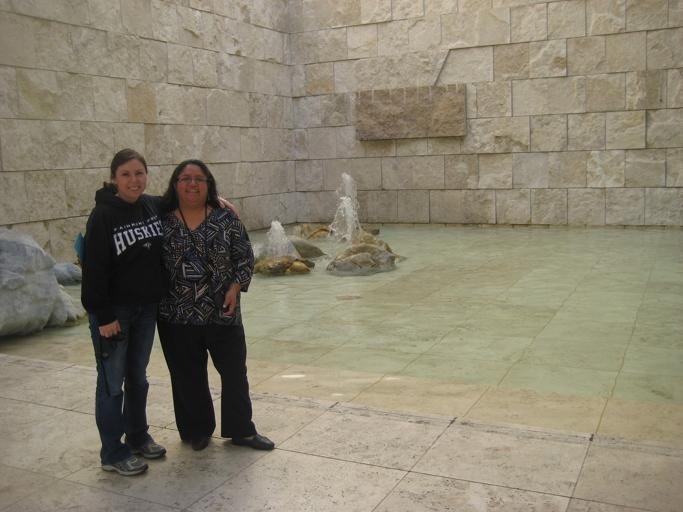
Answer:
[191,433,209,450]
[232,434,274,450]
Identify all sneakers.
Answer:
[124,433,166,459]
[102,454,148,475]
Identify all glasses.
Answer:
[175,176,207,183]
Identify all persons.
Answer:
[80,148,240,476]
[159,159,274,451]
